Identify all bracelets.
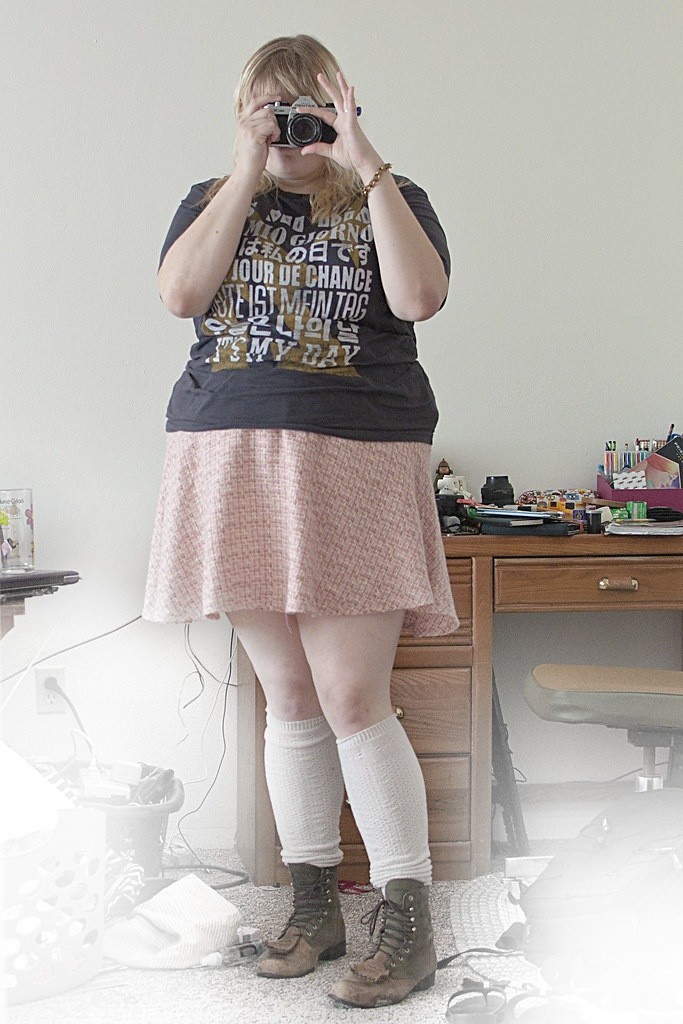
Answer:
[360,162,392,195]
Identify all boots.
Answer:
[255,863,346,978]
[328,878,437,1008]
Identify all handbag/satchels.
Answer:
[99,874,242,970]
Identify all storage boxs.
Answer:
[87,778,184,877]
[597,474,683,513]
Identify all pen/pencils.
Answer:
[665,424,675,443]
[616,519,656,524]
[605,438,649,478]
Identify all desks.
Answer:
[235,530,683,887]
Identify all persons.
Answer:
[140,33,460,1008]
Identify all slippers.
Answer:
[445,987,557,1024]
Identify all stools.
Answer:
[525,663,683,792]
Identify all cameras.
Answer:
[263,95,338,147]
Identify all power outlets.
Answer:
[32,667,68,715]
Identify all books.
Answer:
[472,508,580,536]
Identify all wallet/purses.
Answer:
[646,508,683,522]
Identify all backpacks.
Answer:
[497,788,682,996]
